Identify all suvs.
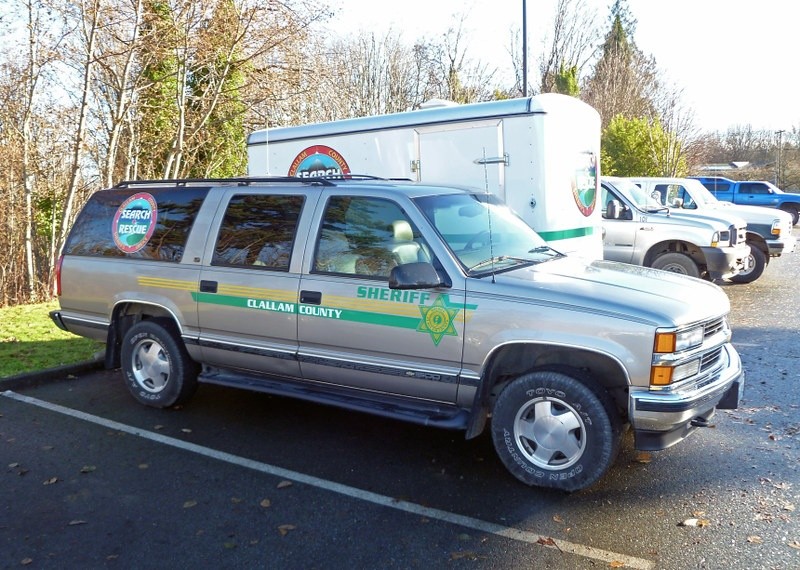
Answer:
[622,177,795,259]
[601,175,751,283]
[48,173,745,493]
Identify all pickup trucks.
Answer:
[675,176,800,228]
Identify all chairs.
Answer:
[606,200,620,219]
[314,230,367,275]
[386,220,429,266]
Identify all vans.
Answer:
[693,161,750,173]
